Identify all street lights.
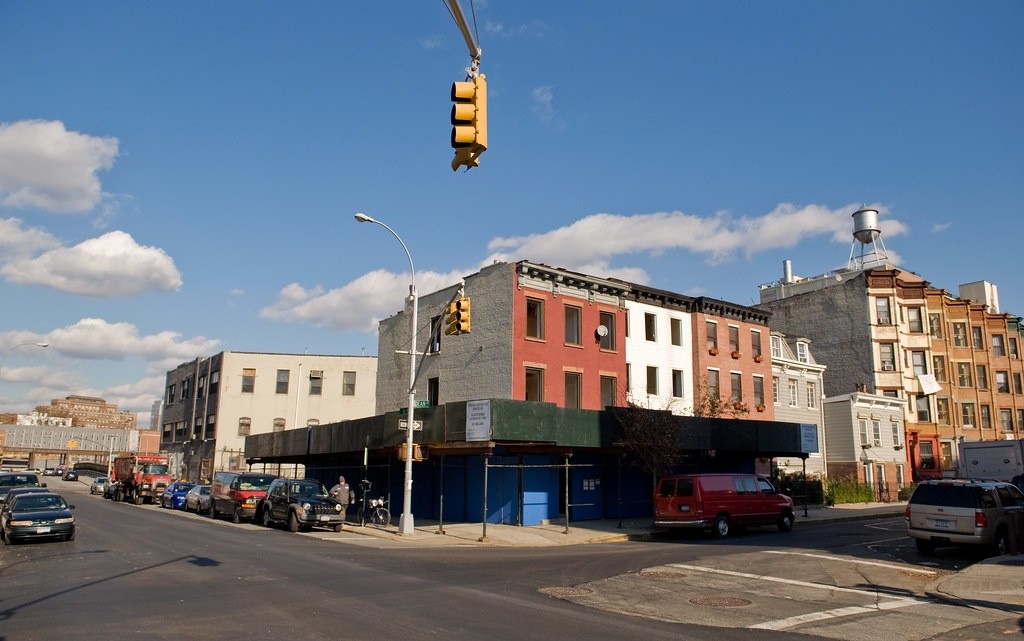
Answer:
[75,434,115,477]
[354,213,418,537]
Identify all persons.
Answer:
[330,476,355,527]
[783,476,793,496]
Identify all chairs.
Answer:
[25,476,33,483]
[304,488,313,496]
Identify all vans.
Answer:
[208,471,278,523]
[21,467,41,475]
[905,478,1024,558]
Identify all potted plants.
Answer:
[710,398,720,406]
[863,444,872,449]
[894,444,904,450]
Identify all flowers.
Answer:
[755,402,767,408]
[754,355,762,358]
[732,351,739,354]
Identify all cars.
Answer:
[0,493,76,545]
[52,468,66,476]
[42,467,55,476]
[159,481,196,511]
[183,485,213,515]
[89,455,172,505]
[0,472,51,535]
[62,471,78,481]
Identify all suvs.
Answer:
[261,478,344,533]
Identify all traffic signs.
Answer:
[398,419,423,431]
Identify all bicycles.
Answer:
[357,494,392,528]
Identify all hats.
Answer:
[339,476,345,482]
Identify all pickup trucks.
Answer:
[654,472,795,541]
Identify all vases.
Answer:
[732,354,741,358]
[734,401,747,409]
[754,357,763,361]
[757,407,766,411]
[709,347,719,354]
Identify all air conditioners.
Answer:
[879,318,889,324]
[192,434,196,439]
[310,370,321,377]
[882,365,893,371]
[1010,353,1016,359]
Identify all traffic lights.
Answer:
[455,297,471,334]
[450,76,487,154]
[445,303,457,336]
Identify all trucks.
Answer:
[958,435,1024,491]
